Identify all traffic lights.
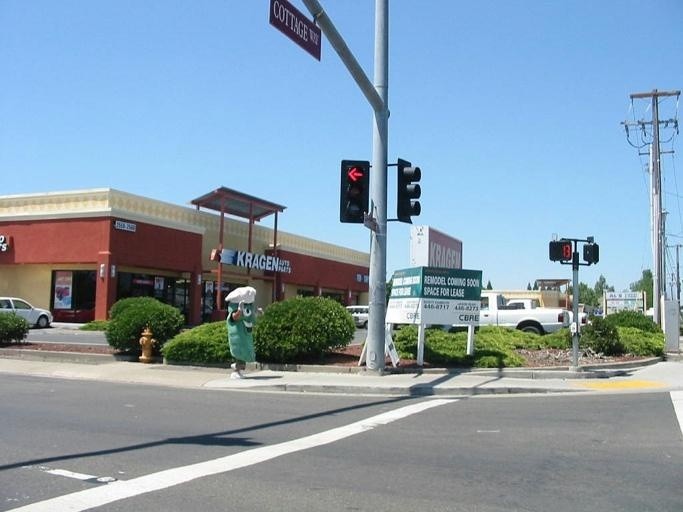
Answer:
[394,157,421,224]
[582,242,599,266]
[337,158,371,224]
[547,240,572,263]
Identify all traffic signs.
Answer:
[267,0,322,62]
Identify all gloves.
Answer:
[256,306,264,316]
[238,301,244,311]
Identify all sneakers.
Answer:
[231,363,238,371]
[231,372,246,379]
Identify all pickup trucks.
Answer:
[451,291,574,335]
[504,299,589,332]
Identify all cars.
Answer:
[0,297,53,330]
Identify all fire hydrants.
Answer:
[135,326,159,364]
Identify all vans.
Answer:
[343,304,368,328]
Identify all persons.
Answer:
[223,285,263,379]
